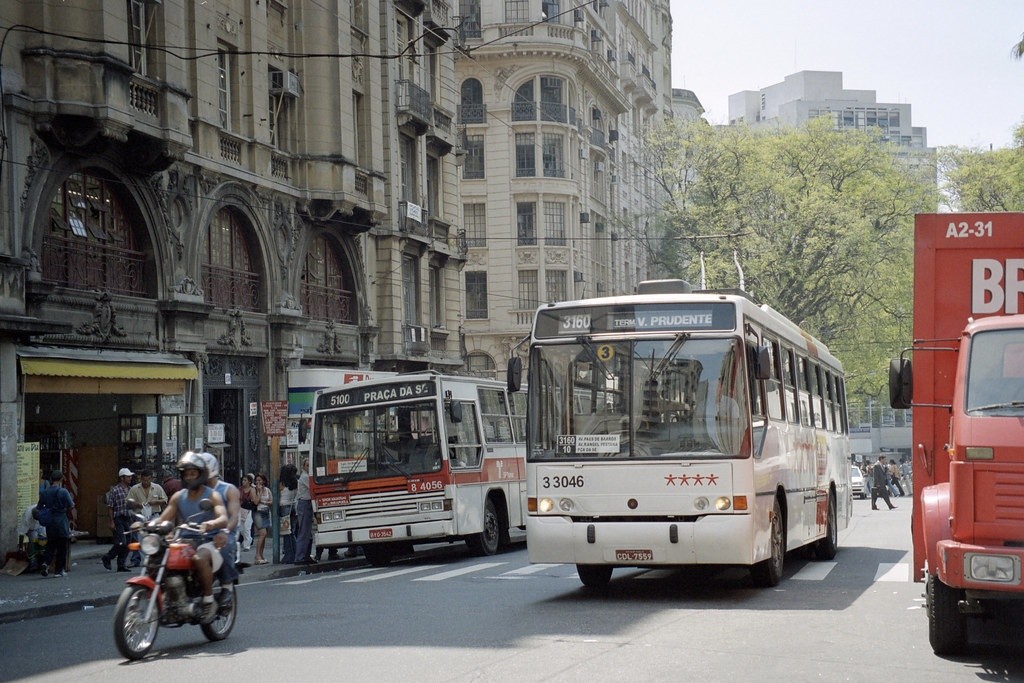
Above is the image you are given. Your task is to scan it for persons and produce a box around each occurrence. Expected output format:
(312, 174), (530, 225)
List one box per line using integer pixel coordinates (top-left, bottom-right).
(239, 458), (364, 565)
(162, 470), (183, 500)
(166, 451), (245, 608)
(102, 468), (135, 572)
(871, 454), (898, 510)
(855, 458), (913, 496)
(18, 466), (75, 578)
(125, 470), (168, 568)
(692, 372), (739, 449)
(130, 451), (229, 624)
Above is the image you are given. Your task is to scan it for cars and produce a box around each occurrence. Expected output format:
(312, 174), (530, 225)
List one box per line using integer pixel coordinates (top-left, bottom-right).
(892, 474), (913, 496)
(851, 465), (867, 499)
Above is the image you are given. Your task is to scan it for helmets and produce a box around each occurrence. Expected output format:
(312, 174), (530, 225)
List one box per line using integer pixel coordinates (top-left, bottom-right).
(174, 451), (209, 490)
(199, 453), (220, 479)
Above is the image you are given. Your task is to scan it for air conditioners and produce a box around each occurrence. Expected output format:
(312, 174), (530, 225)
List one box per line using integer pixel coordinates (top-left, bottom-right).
(597, 282), (607, 292)
(574, 271), (584, 282)
(595, 161), (605, 171)
(611, 175), (619, 183)
(580, 148), (589, 158)
(575, 9), (585, 21)
(270, 70), (300, 97)
(595, 222), (604, 233)
(612, 233), (618, 241)
(607, 49), (618, 62)
(593, 109), (602, 119)
(581, 213), (590, 223)
(610, 130), (618, 142)
(590, 29), (602, 39)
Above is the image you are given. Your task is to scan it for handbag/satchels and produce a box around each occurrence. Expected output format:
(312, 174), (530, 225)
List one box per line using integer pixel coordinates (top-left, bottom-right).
(279, 514), (292, 536)
(141, 503), (152, 521)
(241, 487), (256, 510)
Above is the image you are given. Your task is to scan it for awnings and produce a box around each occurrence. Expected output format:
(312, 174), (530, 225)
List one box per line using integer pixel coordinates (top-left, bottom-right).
(16, 345), (199, 380)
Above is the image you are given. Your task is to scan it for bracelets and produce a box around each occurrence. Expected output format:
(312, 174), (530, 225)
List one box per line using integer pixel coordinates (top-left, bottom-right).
(20, 548), (23, 551)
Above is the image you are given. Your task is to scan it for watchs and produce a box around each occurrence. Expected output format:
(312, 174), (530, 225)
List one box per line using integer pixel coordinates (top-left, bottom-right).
(221, 527), (230, 534)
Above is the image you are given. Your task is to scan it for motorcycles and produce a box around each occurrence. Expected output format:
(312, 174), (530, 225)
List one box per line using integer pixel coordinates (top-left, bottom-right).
(111, 498), (237, 660)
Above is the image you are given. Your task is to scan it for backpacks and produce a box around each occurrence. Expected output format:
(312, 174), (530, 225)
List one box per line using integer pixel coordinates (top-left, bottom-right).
(37, 487), (61, 527)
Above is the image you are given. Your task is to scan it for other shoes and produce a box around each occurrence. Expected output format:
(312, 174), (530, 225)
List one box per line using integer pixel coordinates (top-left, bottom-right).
(128, 563), (141, 568)
(328, 554), (345, 560)
(255, 558), (270, 564)
(53, 569), (68, 577)
(294, 557), (318, 564)
(313, 556), (321, 561)
(117, 566), (132, 572)
(102, 555), (112, 570)
(40, 563), (49, 577)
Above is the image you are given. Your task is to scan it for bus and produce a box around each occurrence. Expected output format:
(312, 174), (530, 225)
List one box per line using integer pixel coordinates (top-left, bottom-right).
(309, 370), (613, 555)
(506, 278), (853, 587)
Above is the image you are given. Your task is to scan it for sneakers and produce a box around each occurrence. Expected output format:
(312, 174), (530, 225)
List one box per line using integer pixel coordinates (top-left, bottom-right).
(216, 587), (232, 607)
(199, 600), (218, 625)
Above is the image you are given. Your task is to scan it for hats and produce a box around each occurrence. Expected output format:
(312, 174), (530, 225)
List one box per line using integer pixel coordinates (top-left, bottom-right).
(118, 468), (135, 477)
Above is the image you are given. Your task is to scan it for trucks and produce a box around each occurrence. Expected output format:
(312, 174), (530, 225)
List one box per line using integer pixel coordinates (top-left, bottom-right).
(889, 212), (1024, 653)
